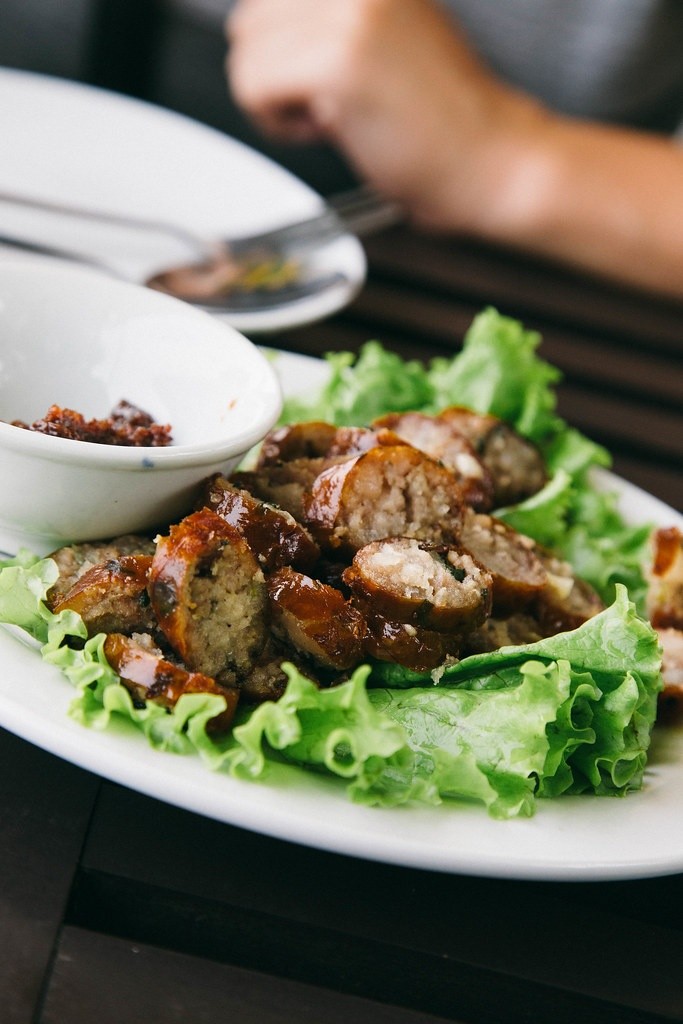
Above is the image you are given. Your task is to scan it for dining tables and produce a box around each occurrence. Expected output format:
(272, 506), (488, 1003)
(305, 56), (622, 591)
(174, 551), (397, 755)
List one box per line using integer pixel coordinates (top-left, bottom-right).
(0, 216), (683, 1024)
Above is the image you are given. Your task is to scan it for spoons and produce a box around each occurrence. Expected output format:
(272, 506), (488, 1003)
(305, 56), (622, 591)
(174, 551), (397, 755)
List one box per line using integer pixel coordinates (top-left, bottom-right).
(0, 235), (348, 309)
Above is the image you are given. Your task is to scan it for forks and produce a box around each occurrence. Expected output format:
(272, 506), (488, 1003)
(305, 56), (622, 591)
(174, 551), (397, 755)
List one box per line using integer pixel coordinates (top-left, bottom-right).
(6, 179), (405, 263)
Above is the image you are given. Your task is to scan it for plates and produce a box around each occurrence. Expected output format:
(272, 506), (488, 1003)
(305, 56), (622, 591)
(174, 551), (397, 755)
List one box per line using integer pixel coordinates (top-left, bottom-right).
(2, 68), (367, 335)
(2, 347), (682, 887)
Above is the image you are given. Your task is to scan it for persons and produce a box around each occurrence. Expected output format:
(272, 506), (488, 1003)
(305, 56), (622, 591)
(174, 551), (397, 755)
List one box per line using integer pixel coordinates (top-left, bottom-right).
(0, 0), (683, 296)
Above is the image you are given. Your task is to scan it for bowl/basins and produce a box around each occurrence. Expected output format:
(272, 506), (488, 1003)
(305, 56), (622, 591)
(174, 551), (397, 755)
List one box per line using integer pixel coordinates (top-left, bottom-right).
(1, 264), (287, 556)
